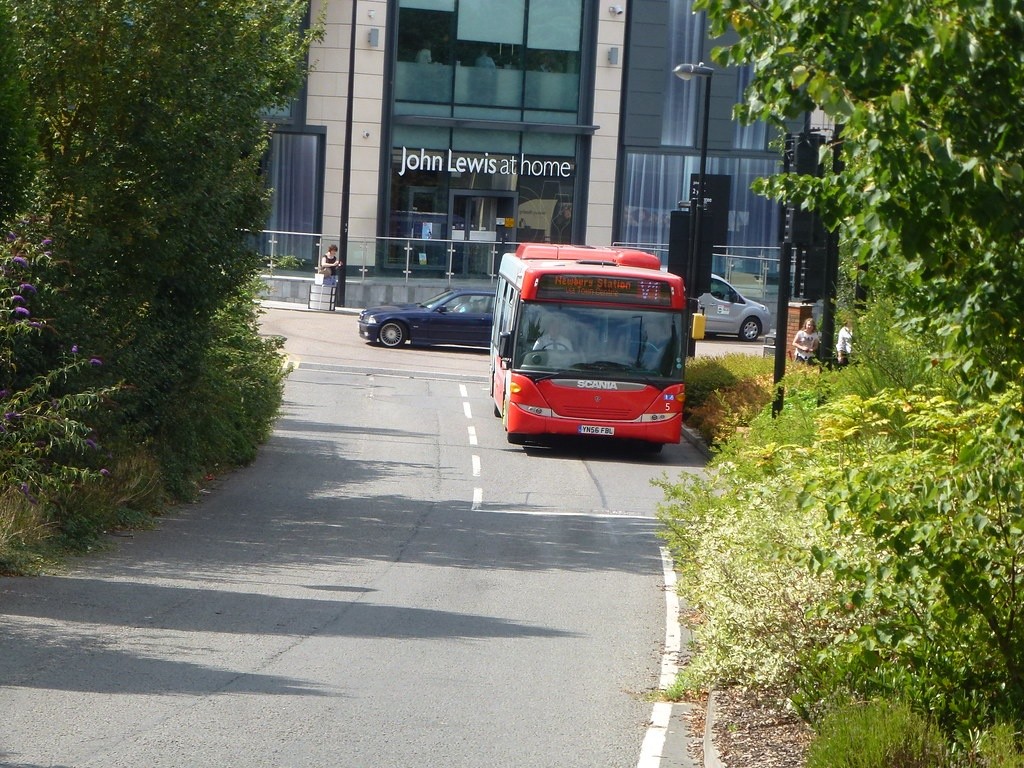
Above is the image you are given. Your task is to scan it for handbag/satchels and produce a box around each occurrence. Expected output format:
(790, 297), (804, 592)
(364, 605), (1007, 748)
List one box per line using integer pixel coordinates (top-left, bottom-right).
(318, 255), (332, 278)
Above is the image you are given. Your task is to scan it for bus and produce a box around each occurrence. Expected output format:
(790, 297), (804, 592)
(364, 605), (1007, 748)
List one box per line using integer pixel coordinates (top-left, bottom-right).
(489, 243), (706, 444)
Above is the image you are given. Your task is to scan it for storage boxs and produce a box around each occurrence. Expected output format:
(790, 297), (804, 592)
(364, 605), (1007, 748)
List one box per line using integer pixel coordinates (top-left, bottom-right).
(308, 301), (336, 311)
(309, 293), (336, 302)
(310, 284), (336, 295)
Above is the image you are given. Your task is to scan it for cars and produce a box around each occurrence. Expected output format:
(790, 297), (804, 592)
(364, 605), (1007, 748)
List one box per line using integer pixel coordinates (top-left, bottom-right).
(358, 288), (496, 350)
(660, 267), (771, 341)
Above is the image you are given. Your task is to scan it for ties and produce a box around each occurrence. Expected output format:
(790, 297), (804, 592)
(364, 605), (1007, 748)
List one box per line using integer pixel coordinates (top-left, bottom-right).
(552, 338), (558, 351)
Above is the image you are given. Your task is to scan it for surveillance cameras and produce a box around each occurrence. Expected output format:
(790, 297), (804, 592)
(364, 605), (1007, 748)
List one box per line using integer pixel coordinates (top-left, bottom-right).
(608, 3), (623, 14)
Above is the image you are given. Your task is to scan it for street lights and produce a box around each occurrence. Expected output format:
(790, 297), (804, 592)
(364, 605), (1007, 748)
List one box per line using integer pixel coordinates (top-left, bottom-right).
(671, 62), (715, 358)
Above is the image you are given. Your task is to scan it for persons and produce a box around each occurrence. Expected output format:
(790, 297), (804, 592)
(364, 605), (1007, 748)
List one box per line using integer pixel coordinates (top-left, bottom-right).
(791, 318), (818, 359)
(533, 320), (574, 352)
(443, 295), (472, 312)
(836, 322), (853, 368)
(322, 244), (344, 307)
